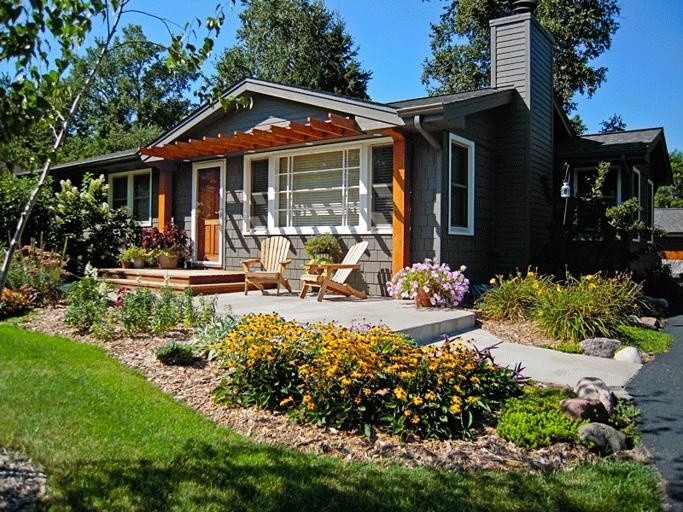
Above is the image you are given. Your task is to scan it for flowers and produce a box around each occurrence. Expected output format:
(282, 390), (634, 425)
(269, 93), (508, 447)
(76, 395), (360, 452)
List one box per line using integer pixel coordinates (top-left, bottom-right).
(385, 257), (471, 307)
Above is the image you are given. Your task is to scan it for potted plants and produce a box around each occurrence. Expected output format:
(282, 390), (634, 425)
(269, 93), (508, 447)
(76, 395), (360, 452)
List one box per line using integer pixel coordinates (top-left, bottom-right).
(305, 235), (342, 260)
(121, 239), (181, 268)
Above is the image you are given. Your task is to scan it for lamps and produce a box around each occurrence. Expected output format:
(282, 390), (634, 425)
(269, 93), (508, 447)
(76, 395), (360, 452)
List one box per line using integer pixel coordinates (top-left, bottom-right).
(561, 161), (572, 199)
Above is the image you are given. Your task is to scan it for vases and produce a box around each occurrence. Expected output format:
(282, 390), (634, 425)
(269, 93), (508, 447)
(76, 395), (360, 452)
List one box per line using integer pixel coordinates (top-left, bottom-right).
(414, 287), (438, 308)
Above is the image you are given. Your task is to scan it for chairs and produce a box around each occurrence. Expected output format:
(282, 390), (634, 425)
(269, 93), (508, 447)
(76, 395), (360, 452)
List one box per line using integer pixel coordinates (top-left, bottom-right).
(300, 240), (369, 302)
(240, 235), (292, 297)
